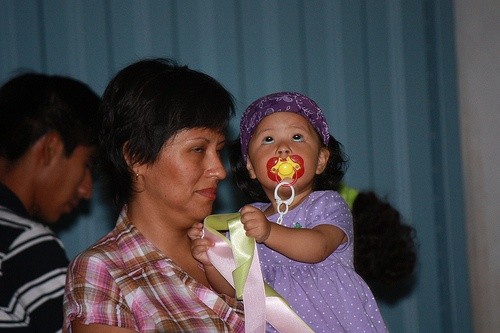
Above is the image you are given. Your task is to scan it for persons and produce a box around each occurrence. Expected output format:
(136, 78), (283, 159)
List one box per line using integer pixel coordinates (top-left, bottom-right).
(0, 73), (101, 333)
(187, 91), (387, 333)
(63, 58), (280, 333)
